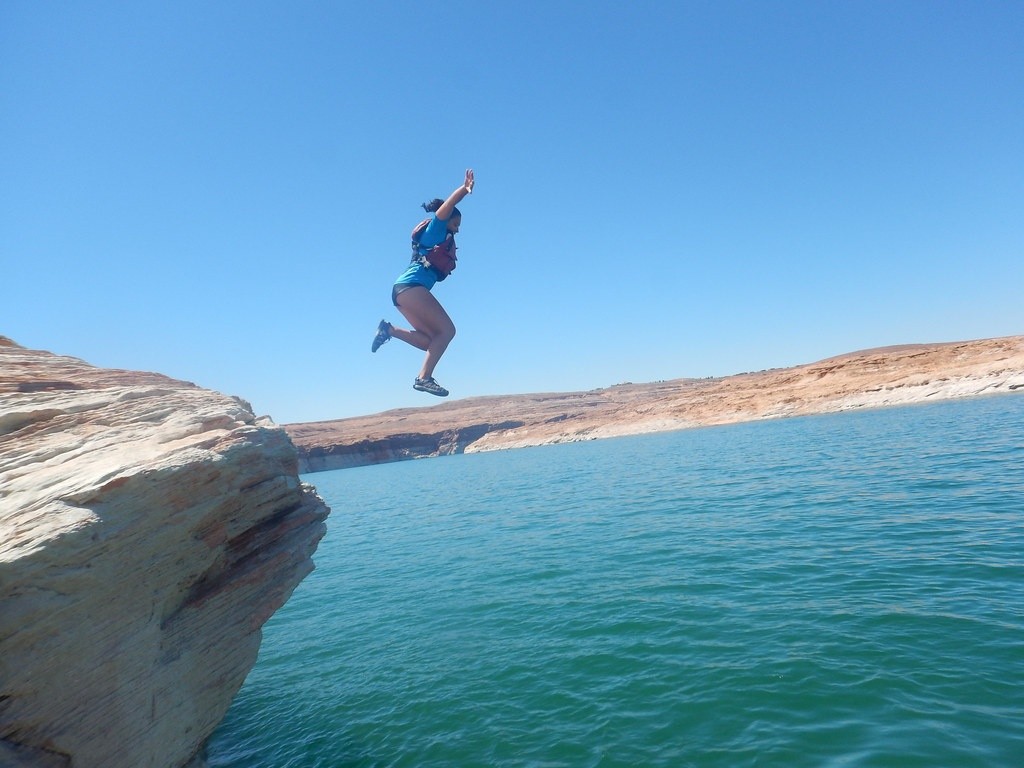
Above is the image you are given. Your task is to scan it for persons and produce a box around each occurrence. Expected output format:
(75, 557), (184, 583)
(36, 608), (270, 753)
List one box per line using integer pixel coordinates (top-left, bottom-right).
(372, 169), (475, 397)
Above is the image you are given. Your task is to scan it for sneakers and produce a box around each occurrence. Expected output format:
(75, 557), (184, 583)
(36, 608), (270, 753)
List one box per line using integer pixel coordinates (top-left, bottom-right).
(371, 320), (392, 352)
(413, 377), (449, 396)
